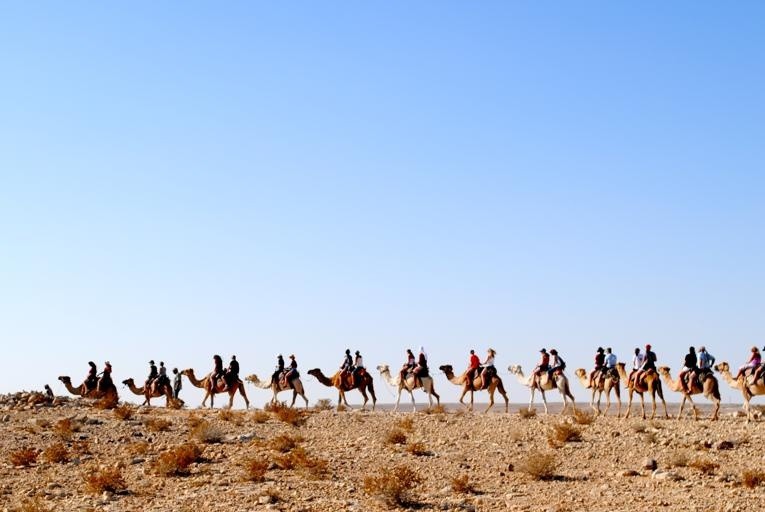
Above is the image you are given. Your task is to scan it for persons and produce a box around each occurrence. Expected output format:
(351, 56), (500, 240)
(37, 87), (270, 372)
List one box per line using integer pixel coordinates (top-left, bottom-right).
(44, 384), (54, 399)
